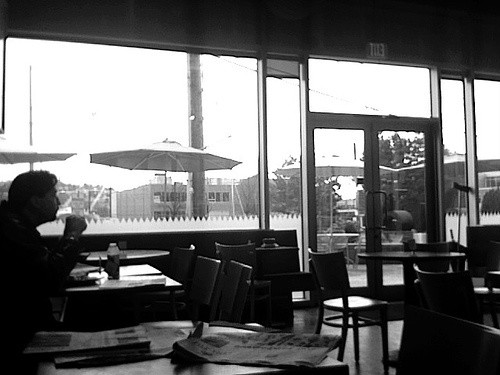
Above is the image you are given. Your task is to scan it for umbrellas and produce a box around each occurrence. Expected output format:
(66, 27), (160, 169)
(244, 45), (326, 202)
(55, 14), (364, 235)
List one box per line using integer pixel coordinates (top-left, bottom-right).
(90, 137), (243, 220)
(397, 150), (500, 243)
(272, 150), (397, 235)
(0, 128), (79, 167)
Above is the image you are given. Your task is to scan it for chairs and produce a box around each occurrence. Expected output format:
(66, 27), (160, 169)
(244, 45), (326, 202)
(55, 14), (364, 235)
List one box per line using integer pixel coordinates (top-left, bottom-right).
(308, 248), (390, 371)
(218, 260), (252, 324)
(397, 303), (500, 375)
(171, 248), (195, 278)
(193, 255), (222, 320)
(412, 263), (500, 328)
(218, 242), (256, 276)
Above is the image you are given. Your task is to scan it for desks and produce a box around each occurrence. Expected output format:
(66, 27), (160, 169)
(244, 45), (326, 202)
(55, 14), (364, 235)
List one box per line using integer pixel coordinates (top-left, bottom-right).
(86, 251), (170, 263)
(39, 323), (349, 375)
(65, 264), (183, 320)
(356, 251), (467, 308)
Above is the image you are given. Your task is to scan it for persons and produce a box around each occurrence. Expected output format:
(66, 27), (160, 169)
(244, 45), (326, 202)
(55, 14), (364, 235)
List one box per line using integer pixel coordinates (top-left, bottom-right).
(0, 170), (87, 375)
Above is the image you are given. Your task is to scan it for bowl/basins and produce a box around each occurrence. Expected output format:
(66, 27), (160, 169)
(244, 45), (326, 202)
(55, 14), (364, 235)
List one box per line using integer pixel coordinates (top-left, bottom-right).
(262, 238), (275, 245)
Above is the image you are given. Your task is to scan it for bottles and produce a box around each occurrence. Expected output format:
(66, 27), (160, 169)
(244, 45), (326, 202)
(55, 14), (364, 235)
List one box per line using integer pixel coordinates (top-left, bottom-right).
(106, 243), (120, 280)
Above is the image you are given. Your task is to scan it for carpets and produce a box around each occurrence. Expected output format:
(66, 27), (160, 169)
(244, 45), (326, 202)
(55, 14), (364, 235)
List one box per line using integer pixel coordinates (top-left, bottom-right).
(359, 301), (405, 321)
